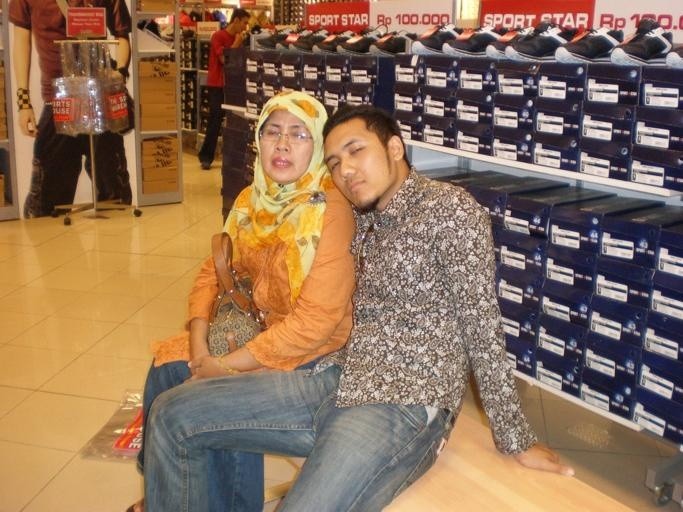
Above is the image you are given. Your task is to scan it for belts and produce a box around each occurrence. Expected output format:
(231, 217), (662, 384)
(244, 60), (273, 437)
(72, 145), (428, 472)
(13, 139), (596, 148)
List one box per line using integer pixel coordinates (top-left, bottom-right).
(445, 409), (457, 425)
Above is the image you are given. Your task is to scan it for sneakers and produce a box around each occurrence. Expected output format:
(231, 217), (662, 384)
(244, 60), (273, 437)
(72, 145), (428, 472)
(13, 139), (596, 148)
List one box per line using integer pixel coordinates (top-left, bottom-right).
(369, 31), (418, 57)
(487, 27), (535, 57)
(555, 26), (624, 63)
(290, 28), (329, 51)
(167, 29), (195, 39)
(336, 26), (383, 54)
(443, 26), (510, 57)
(505, 23), (577, 63)
(312, 30), (358, 53)
(611, 19), (672, 66)
(256, 28), (294, 49)
(665, 47), (683, 68)
(412, 23), (466, 54)
(202, 161), (210, 169)
(276, 30), (313, 50)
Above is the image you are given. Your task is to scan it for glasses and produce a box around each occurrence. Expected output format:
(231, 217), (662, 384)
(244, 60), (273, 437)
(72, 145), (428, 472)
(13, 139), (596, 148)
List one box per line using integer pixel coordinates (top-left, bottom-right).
(260, 128), (313, 143)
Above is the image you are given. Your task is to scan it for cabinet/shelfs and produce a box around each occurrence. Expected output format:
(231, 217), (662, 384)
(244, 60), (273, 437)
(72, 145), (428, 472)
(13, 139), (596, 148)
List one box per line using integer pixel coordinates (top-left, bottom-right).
(180, 1), (224, 160)
(130, 1), (182, 208)
(221, 45), (683, 451)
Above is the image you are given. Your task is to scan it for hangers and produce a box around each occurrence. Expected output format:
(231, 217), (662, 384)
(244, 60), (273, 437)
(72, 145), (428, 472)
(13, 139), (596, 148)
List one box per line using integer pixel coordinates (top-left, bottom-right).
(52, 38), (144, 226)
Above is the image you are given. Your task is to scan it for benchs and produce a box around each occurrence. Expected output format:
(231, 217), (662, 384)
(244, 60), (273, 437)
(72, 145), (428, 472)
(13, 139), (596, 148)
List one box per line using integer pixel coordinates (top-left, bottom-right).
(258, 410), (634, 512)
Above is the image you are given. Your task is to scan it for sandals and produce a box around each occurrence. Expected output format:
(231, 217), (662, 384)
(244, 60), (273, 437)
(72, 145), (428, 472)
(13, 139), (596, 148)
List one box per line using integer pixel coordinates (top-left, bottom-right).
(126, 499), (146, 512)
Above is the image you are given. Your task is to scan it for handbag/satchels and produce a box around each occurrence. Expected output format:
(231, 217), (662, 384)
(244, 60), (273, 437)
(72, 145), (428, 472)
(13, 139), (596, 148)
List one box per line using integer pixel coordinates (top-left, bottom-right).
(51, 43), (128, 136)
(207, 232), (265, 356)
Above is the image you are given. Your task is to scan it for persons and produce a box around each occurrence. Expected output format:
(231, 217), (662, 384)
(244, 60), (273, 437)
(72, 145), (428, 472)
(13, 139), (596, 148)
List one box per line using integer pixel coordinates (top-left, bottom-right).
(198, 8), (251, 170)
(8, 0), (132, 218)
(143, 104), (574, 512)
(128, 90), (357, 511)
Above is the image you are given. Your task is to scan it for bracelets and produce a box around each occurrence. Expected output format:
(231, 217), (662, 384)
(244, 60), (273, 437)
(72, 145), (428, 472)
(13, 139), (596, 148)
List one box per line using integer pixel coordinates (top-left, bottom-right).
(216, 354), (242, 375)
